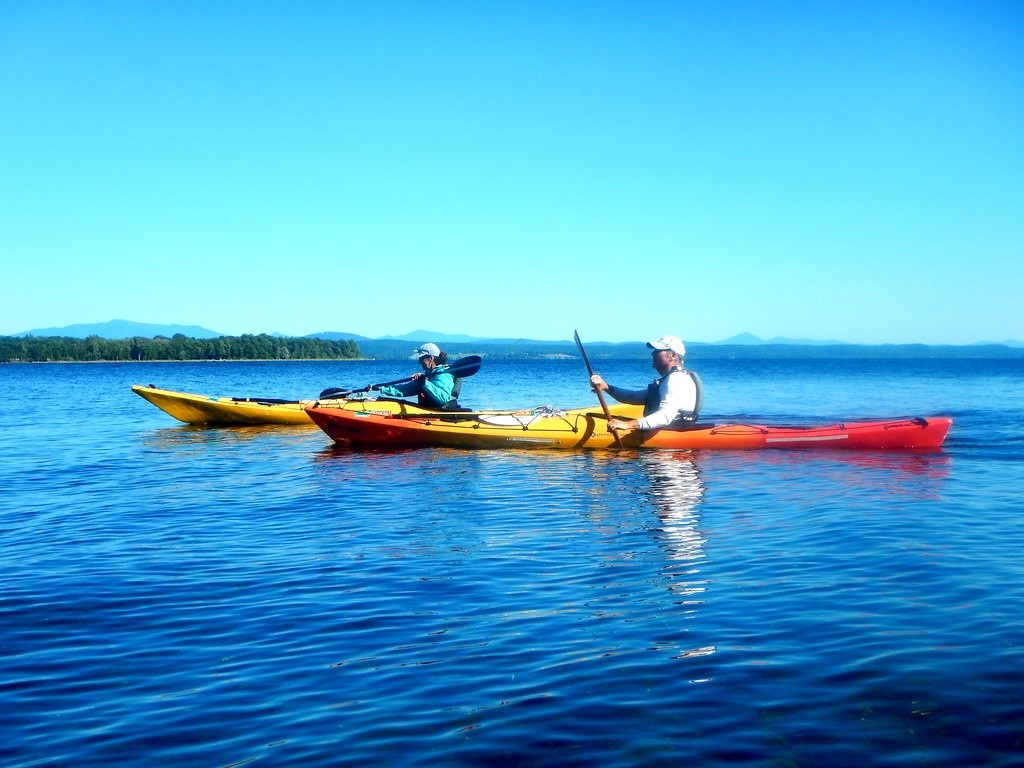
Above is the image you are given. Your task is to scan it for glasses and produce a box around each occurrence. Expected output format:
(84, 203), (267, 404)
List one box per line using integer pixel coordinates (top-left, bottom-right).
(417, 349), (430, 354)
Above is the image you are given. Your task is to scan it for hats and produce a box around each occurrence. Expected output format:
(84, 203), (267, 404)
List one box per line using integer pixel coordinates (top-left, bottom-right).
(410, 343), (441, 359)
(646, 336), (686, 357)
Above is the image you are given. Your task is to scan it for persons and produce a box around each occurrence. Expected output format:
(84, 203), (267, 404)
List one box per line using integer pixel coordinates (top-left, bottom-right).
(590, 335), (696, 433)
(369, 343), (462, 409)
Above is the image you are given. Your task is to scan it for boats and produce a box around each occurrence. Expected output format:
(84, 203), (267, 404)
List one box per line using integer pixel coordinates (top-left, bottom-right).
(302, 400), (953, 452)
(132, 385), (645, 426)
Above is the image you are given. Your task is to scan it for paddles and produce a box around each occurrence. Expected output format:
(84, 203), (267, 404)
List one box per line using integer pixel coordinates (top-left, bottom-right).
(319, 355), (481, 401)
(573, 329), (624, 450)
